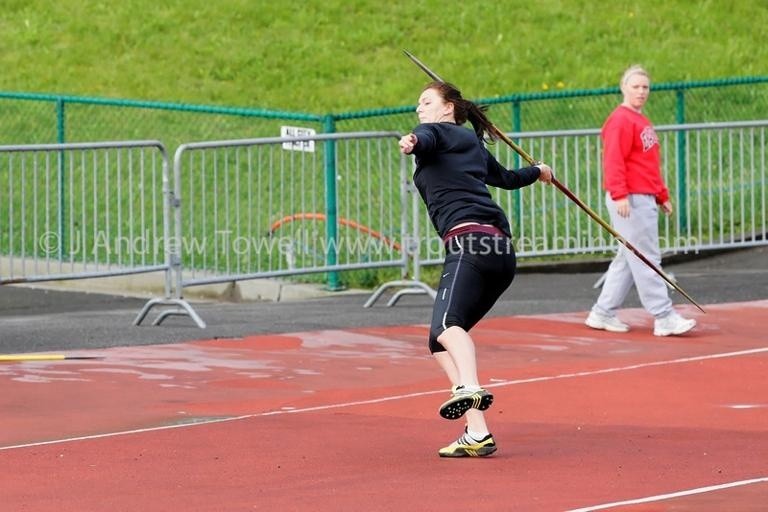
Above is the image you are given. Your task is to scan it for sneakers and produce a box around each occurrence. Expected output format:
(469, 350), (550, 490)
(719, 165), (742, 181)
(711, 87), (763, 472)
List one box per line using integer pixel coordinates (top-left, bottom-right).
(438, 423), (497, 456)
(585, 311), (630, 334)
(437, 384), (494, 419)
(652, 310), (697, 337)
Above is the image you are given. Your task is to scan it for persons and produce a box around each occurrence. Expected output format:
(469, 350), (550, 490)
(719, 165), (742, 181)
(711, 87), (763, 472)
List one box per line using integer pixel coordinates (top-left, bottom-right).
(583, 62), (698, 337)
(396, 79), (556, 460)
